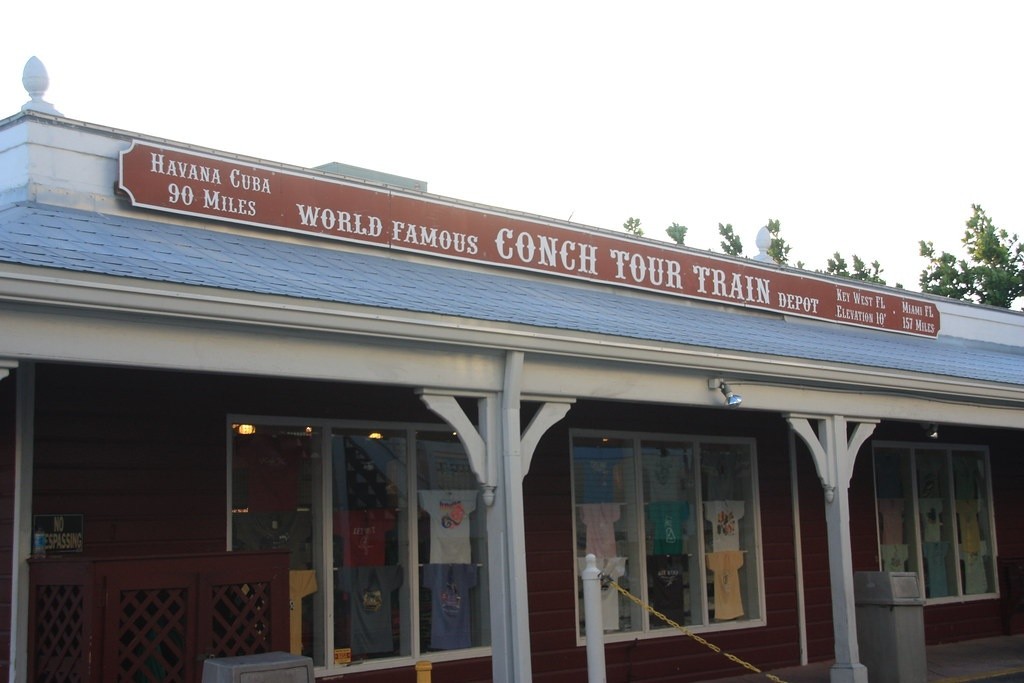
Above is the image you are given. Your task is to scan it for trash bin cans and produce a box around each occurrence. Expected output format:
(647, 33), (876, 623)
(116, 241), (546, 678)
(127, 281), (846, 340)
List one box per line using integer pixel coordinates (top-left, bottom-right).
(200, 650), (316, 683)
(854, 570), (929, 683)
